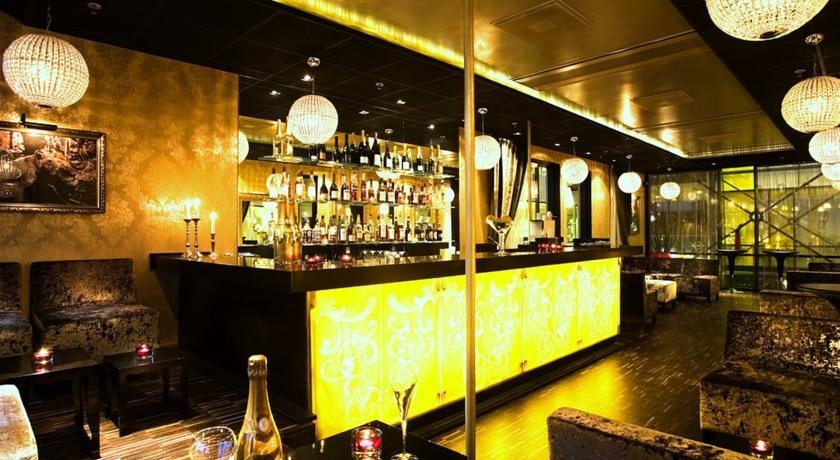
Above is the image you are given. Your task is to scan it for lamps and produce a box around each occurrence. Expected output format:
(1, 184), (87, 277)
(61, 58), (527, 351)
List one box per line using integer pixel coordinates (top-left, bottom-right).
(560, 138), (589, 185)
(618, 154), (642, 194)
(781, 33), (840, 190)
(705, 0), (829, 41)
(474, 107), (501, 171)
(288, 56), (338, 147)
(658, 167), (681, 200)
(2, 7), (90, 109)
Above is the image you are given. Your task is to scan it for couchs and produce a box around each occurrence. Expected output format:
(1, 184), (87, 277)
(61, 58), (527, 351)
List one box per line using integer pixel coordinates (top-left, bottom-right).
(0, 258), (162, 372)
(547, 256), (840, 460)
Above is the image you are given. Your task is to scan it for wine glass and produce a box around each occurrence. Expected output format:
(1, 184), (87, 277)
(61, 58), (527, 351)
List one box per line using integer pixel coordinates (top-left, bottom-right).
(389, 361), (419, 460)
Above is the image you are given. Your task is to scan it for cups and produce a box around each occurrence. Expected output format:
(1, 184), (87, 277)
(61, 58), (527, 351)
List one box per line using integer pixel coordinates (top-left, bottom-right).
(749, 436), (774, 460)
(189, 426), (238, 460)
(31, 342), (53, 372)
(350, 426), (383, 460)
(538, 238), (562, 254)
(135, 334), (154, 360)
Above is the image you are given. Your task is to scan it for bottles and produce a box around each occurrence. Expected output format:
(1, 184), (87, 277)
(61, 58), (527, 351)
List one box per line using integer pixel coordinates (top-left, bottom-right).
(273, 193), (302, 266)
(233, 354), (284, 460)
(255, 116), (446, 244)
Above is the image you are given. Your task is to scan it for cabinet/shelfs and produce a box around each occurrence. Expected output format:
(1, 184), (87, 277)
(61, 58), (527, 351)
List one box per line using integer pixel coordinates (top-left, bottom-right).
(238, 155), (455, 259)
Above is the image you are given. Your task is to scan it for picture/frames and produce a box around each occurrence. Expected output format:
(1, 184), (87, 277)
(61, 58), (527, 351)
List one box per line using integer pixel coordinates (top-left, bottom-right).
(0, 121), (106, 215)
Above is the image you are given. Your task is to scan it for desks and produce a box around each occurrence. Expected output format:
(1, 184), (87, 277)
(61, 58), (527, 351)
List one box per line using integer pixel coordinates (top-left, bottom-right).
(0, 344), (193, 460)
(282, 420), (469, 460)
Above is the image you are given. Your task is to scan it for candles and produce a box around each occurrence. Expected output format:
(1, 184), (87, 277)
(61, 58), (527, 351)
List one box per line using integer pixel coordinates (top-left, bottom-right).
(211, 214), (216, 233)
(354, 429), (382, 452)
(136, 345), (153, 357)
(33, 347), (54, 363)
(185, 200), (199, 218)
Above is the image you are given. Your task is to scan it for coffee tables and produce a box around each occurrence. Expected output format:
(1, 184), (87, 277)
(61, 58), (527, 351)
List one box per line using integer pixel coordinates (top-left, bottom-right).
(796, 282), (840, 297)
(715, 248), (748, 294)
(645, 279), (677, 312)
(761, 249), (800, 291)
